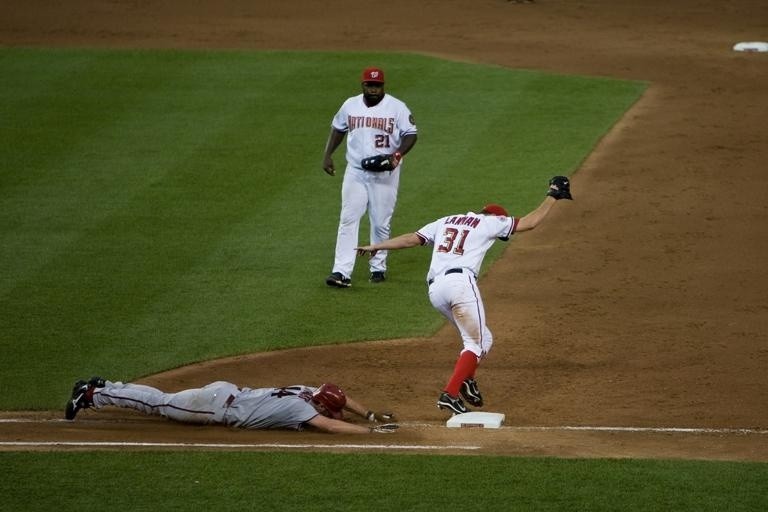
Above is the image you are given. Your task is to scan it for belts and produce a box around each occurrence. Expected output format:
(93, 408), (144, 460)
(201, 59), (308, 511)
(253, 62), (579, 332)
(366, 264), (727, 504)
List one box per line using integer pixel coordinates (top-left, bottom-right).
(429, 268), (462, 285)
(226, 388), (241, 406)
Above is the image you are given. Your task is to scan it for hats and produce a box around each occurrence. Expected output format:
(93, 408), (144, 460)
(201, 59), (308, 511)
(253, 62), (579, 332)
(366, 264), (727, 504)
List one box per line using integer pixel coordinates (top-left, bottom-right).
(361, 68), (384, 83)
(482, 205), (509, 241)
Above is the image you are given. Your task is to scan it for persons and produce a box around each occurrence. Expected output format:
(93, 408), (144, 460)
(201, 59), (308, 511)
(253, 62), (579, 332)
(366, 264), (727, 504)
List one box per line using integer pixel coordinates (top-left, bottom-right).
(64, 373), (402, 436)
(353, 173), (574, 414)
(321, 67), (420, 289)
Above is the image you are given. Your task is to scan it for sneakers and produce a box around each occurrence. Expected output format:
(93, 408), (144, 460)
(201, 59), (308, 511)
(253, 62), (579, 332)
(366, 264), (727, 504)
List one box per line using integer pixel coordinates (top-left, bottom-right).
(369, 271), (386, 282)
(327, 272), (352, 287)
(459, 377), (483, 407)
(437, 393), (470, 414)
(65, 381), (93, 421)
(89, 377), (105, 388)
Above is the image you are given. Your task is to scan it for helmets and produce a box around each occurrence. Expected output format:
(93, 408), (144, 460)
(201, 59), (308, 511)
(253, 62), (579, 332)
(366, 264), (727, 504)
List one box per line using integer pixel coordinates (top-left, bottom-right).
(312, 384), (346, 419)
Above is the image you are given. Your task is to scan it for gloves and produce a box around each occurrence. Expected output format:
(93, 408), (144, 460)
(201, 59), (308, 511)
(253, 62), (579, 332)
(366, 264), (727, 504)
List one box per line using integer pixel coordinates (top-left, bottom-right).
(368, 424), (399, 434)
(365, 410), (395, 423)
